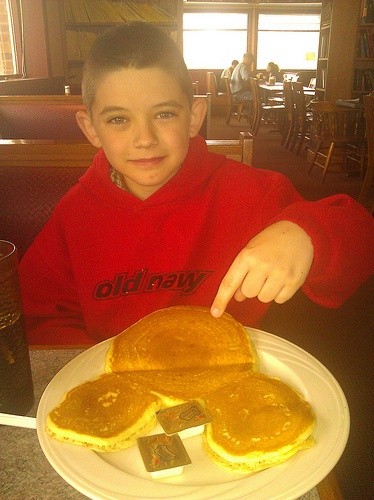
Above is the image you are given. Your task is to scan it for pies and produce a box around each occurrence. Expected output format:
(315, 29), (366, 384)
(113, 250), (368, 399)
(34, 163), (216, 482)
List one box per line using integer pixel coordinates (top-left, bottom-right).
(104, 306), (256, 403)
(203, 373), (317, 466)
(46, 374), (163, 452)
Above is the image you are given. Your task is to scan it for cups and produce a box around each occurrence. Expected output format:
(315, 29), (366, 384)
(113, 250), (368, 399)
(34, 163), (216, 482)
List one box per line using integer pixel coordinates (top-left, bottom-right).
(0, 239), (35, 426)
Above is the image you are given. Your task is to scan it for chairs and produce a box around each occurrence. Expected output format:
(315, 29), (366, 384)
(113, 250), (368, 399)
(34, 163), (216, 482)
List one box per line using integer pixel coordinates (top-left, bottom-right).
(194, 66), (374, 199)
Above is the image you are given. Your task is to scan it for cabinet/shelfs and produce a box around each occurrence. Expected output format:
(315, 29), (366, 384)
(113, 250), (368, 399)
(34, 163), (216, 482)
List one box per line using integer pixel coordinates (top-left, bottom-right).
(316, 0), (360, 100)
(353, 0), (374, 100)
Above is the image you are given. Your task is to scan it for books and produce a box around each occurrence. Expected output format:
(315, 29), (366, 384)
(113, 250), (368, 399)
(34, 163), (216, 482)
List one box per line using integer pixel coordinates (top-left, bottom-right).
(354, 0), (374, 91)
(318, 0), (333, 88)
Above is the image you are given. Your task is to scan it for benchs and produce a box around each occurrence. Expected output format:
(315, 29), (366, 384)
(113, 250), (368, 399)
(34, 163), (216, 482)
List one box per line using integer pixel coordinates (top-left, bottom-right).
(0, 132), (254, 261)
(0, 94), (212, 140)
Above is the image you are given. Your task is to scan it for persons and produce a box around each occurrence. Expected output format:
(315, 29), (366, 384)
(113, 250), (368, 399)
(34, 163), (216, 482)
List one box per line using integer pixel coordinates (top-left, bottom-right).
(220, 52), (283, 120)
(19, 20), (374, 348)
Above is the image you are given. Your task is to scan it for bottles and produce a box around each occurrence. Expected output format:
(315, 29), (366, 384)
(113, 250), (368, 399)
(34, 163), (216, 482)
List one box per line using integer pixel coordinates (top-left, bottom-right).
(64, 85), (71, 96)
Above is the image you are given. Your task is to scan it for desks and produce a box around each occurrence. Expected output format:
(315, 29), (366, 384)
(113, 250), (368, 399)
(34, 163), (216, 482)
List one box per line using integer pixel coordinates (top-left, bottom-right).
(310, 99), (365, 170)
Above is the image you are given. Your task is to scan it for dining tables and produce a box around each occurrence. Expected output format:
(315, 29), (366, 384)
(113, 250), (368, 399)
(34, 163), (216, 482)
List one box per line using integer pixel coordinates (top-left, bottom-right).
(0, 349), (344, 500)
(258, 81), (315, 120)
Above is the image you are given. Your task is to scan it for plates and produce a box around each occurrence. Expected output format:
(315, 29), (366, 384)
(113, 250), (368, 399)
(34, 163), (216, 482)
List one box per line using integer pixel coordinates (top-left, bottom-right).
(35, 327), (350, 500)
